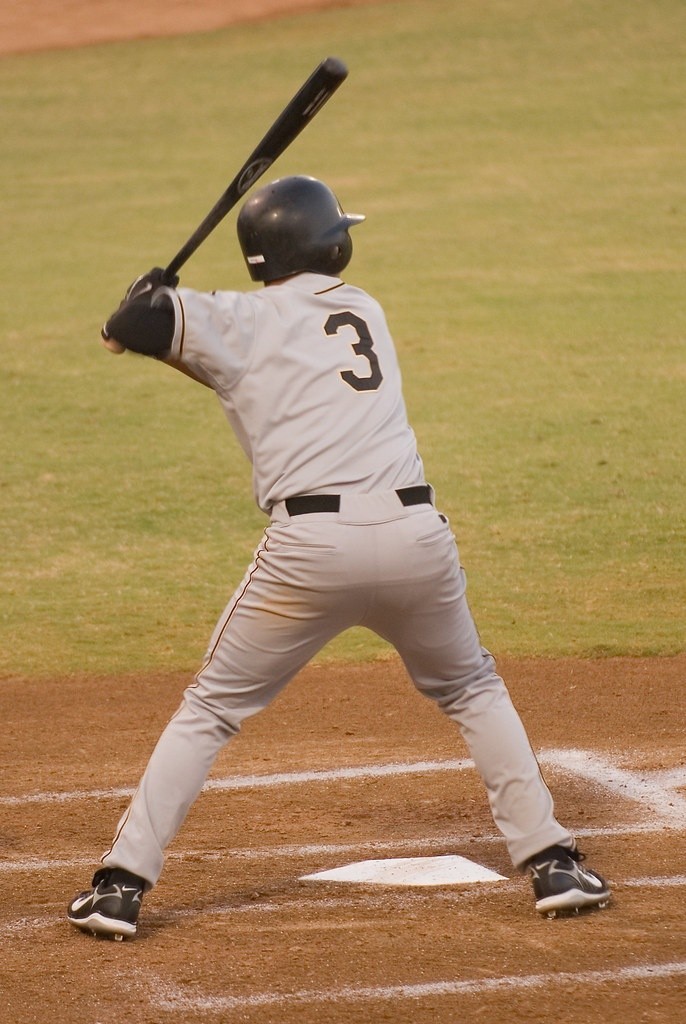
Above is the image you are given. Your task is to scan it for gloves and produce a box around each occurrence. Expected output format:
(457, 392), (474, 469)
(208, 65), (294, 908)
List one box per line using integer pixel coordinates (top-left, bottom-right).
(101, 267), (180, 341)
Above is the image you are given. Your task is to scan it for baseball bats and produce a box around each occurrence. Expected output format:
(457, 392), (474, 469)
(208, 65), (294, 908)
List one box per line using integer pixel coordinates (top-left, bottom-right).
(101, 57), (351, 355)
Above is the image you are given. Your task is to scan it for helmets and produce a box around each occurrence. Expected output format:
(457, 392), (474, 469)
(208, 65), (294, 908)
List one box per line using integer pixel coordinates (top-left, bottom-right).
(237, 175), (366, 281)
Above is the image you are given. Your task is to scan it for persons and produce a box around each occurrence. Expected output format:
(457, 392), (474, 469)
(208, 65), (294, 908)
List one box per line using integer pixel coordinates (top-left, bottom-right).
(69, 175), (611, 943)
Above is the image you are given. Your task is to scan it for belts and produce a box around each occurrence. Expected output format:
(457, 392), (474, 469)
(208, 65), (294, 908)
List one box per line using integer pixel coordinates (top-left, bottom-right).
(268, 486), (433, 516)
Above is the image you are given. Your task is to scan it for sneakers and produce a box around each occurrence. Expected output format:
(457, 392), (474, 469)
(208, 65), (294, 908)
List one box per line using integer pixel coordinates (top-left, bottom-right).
(532, 845), (611, 918)
(67, 868), (143, 941)
